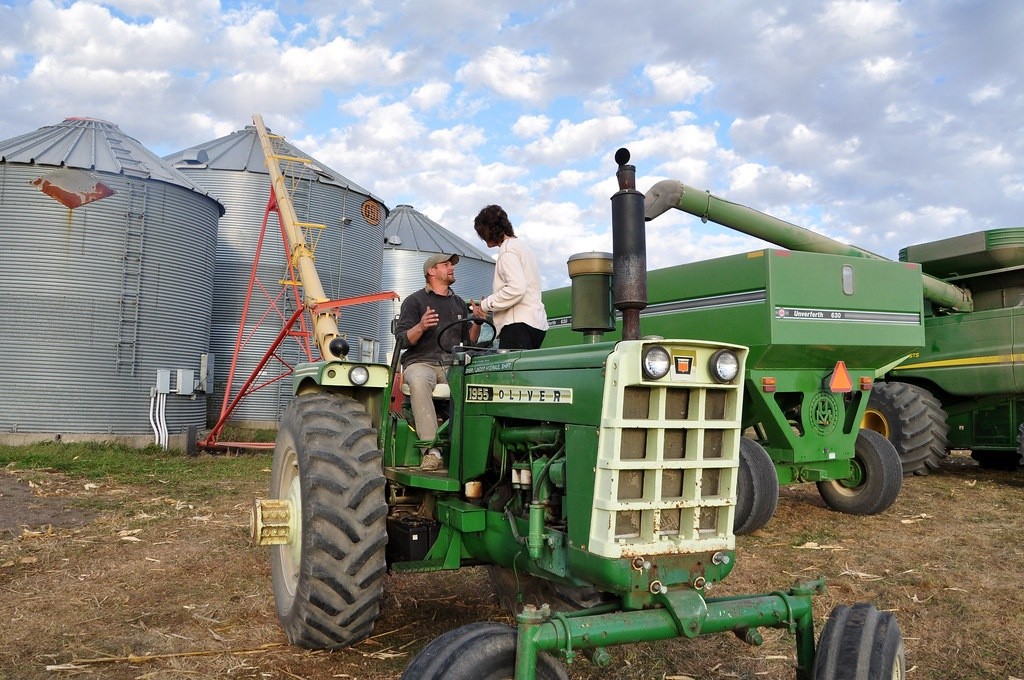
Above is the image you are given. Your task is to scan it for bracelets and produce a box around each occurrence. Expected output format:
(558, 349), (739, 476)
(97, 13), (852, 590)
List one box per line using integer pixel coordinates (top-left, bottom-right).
(473, 315), (483, 325)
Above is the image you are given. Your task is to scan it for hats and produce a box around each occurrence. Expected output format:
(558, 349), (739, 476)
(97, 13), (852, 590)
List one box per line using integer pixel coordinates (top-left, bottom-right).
(423, 253), (460, 277)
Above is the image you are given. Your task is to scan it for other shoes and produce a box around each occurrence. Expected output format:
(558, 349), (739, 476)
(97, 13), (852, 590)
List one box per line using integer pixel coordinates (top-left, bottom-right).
(420, 452), (444, 470)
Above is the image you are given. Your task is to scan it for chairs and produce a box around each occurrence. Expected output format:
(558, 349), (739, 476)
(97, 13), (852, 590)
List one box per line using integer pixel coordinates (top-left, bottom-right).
(391, 312), (453, 401)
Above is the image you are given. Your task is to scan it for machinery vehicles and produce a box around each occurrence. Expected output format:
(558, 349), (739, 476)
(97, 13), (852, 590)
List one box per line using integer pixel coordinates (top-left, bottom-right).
(250, 147), (908, 680)
(645, 179), (1024, 477)
(541, 250), (925, 536)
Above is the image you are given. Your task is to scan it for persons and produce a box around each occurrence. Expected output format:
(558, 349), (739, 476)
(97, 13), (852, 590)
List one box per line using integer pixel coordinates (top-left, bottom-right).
(395, 254), (487, 471)
(474, 205), (550, 352)
